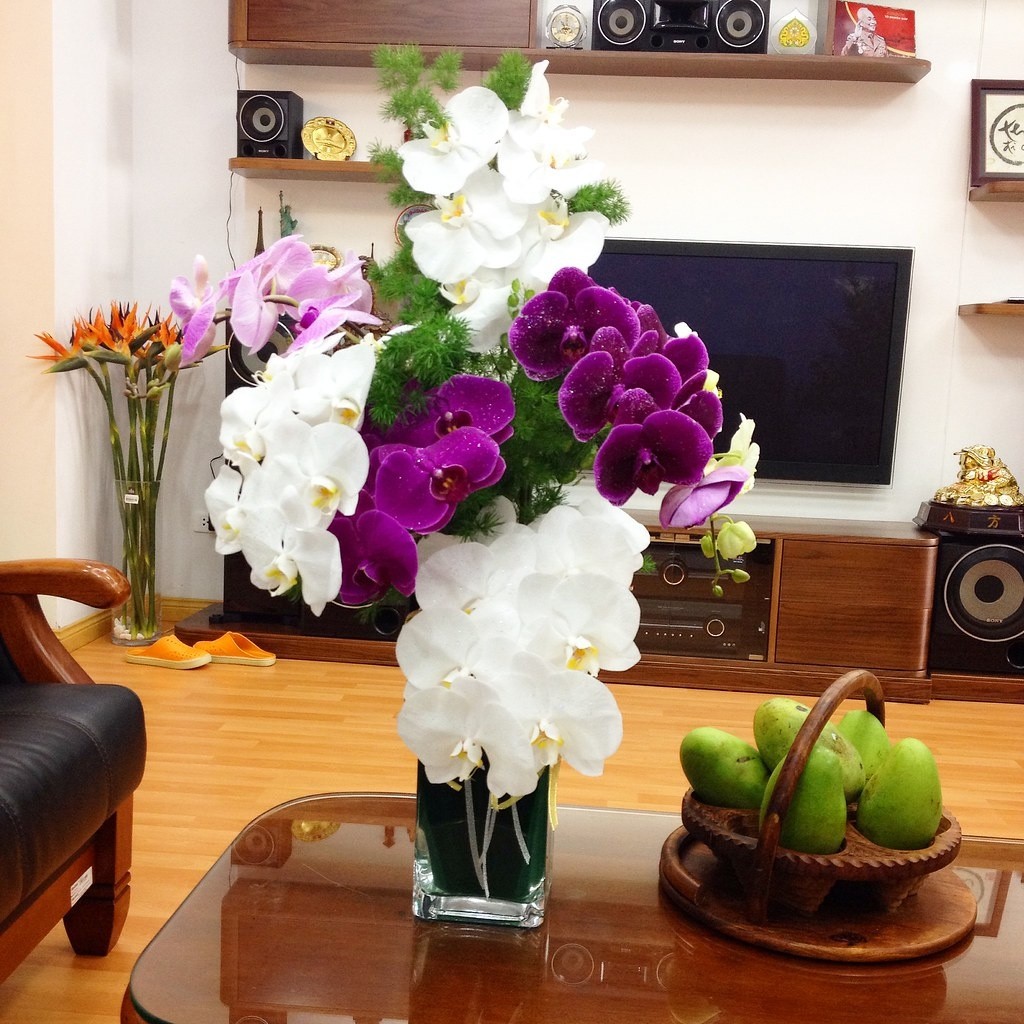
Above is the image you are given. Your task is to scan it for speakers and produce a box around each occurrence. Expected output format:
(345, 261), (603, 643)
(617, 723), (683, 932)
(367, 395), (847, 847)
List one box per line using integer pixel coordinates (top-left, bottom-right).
(298, 597), (415, 642)
(712, 0), (771, 55)
(926, 527), (1024, 677)
(236, 89), (306, 161)
(592, 0), (652, 51)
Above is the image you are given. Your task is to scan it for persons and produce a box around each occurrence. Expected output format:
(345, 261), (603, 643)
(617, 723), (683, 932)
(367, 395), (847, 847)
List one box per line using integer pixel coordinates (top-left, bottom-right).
(840, 8), (888, 58)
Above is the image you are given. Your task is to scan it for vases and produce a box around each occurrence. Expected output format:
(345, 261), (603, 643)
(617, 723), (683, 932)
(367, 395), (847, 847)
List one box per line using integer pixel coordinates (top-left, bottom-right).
(412, 753), (555, 933)
(111, 479), (161, 646)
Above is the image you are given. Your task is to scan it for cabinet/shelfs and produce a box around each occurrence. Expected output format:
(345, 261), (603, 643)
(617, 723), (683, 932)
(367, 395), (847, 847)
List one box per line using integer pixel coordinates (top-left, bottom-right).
(171, 508), (1024, 705)
(226, 1), (1024, 317)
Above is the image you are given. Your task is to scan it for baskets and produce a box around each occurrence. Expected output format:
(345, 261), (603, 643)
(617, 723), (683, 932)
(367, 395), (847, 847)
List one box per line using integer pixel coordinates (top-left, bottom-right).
(681, 668), (962, 919)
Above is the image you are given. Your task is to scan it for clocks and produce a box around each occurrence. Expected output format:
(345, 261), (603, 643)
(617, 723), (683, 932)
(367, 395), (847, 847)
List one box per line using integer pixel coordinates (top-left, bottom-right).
(544, 4), (587, 50)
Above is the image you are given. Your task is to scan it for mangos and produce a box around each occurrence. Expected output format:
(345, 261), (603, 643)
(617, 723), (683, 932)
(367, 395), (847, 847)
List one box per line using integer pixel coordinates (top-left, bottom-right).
(679, 697), (942, 855)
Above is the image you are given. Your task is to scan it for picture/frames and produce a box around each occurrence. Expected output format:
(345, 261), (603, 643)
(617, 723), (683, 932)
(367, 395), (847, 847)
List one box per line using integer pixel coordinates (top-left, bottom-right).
(971, 78), (1024, 187)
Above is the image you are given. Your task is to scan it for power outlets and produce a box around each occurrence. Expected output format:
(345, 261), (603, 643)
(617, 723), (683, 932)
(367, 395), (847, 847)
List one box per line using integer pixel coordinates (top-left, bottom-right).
(193, 512), (215, 533)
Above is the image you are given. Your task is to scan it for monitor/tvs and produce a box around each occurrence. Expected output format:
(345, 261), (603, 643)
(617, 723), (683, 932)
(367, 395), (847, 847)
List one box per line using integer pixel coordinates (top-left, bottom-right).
(589, 236), (916, 489)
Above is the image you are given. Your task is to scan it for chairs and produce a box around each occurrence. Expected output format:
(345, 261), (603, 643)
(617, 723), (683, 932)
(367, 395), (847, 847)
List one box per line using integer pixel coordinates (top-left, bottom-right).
(0, 559), (147, 985)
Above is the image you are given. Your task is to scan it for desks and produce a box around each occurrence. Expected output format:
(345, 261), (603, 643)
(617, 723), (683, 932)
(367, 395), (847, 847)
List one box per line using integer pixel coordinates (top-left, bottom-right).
(121, 792), (1024, 1024)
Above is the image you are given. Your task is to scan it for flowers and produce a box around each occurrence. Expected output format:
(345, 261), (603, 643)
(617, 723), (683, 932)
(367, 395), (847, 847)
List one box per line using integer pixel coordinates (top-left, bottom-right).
(26, 301), (228, 639)
